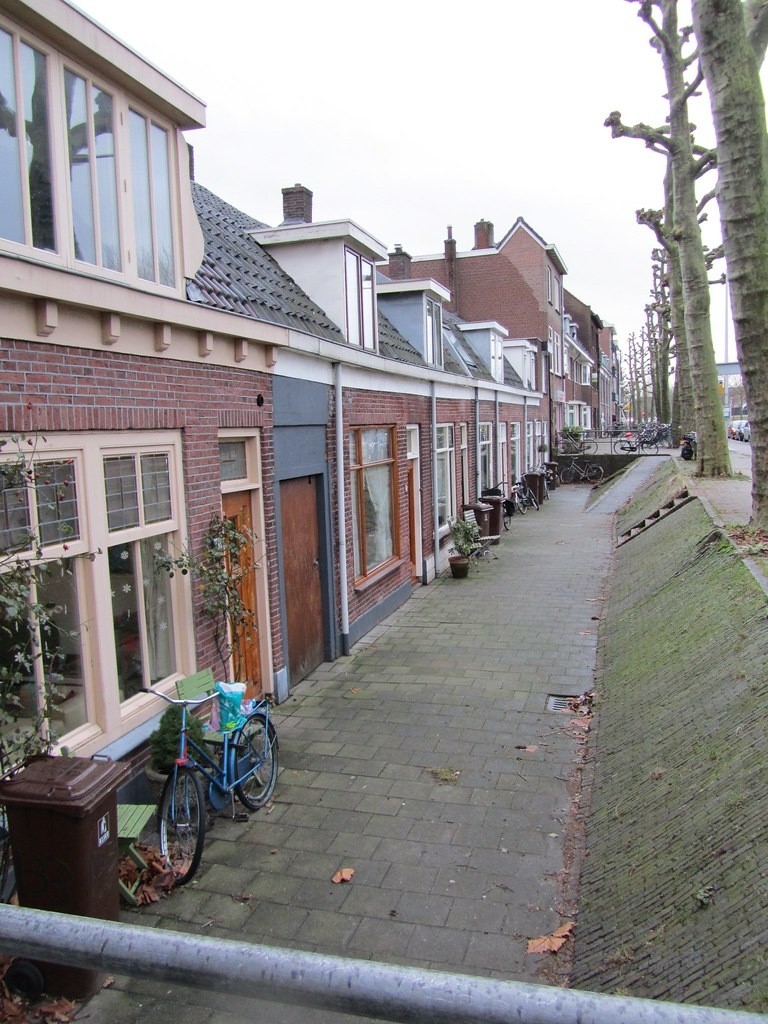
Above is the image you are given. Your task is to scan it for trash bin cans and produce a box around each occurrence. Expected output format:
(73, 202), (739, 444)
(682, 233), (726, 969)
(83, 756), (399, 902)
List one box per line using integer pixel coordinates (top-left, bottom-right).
(0, 751), (133, 1002)
(543, 462), (558, 490)
(522, 472), (545, 505)
(460, 503), (494, 550)
(478, 495), (506, 545)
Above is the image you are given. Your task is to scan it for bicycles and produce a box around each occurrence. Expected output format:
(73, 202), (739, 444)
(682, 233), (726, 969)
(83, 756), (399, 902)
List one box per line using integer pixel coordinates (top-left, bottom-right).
(560, 456), (604, 484)
(137, 681), (280, 887)
(482, 472), (539, 531)
(556, 419), (673, 454)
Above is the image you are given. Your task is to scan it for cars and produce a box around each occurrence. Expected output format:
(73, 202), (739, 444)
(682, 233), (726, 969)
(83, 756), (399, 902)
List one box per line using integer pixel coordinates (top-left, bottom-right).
(727, 420), (751, 442)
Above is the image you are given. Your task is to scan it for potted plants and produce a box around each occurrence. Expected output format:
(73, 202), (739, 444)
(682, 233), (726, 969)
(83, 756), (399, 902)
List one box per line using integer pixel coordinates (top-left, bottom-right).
(447, 515), (482, 579)
(144, 703), (221, 810)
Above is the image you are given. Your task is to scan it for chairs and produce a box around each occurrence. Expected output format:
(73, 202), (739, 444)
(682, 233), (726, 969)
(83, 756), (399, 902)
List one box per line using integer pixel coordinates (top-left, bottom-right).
(447, 510), (501, 573)
(176, 668), (265, 802)
(60, 746), (159, 906)
